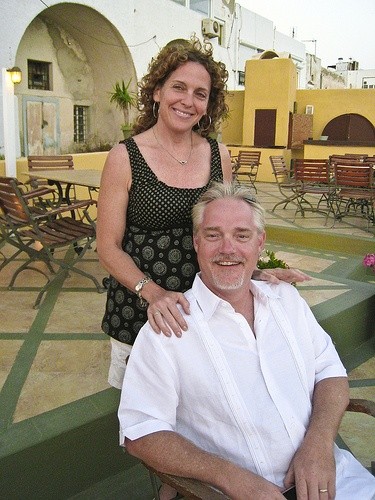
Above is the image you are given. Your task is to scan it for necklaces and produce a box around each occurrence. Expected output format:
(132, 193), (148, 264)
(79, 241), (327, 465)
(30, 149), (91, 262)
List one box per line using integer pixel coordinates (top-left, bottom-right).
(152, 126), (194, 166)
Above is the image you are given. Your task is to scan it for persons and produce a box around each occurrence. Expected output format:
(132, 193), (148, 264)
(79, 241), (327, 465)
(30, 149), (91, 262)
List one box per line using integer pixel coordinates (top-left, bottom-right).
(117, 179), (375, 500)
(96, 36), (312, 500)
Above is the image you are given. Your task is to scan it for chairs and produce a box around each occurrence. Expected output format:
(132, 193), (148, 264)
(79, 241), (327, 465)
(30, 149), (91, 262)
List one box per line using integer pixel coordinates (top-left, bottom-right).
(269, 152), (375, 233)
(228, 149), (261, 194)
(0, 155), (105, 310)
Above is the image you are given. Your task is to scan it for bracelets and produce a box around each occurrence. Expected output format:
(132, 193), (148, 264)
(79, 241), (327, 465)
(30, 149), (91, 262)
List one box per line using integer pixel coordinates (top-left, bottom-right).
(135, 276), (153, 308)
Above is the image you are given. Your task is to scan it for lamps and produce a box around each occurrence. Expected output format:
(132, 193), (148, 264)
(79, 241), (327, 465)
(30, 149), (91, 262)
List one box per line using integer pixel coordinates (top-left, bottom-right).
(6, 66), (23, 85)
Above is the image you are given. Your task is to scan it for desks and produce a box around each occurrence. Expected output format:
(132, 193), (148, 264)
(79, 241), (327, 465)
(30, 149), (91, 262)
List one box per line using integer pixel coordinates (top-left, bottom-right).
(21, 168), (103, 256)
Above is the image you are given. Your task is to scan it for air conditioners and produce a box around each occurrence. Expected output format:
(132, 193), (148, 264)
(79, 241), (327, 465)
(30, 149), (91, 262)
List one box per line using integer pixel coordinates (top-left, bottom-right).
(202, 18), (220, 39)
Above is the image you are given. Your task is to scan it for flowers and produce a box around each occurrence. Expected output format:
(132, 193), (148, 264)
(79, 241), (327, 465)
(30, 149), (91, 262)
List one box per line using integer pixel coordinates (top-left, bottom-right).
(363, 253), (375, 271)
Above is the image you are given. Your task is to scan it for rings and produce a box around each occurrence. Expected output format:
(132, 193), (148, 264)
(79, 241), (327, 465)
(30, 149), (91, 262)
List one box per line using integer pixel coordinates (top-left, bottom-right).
(319, 488), (328, 493)
